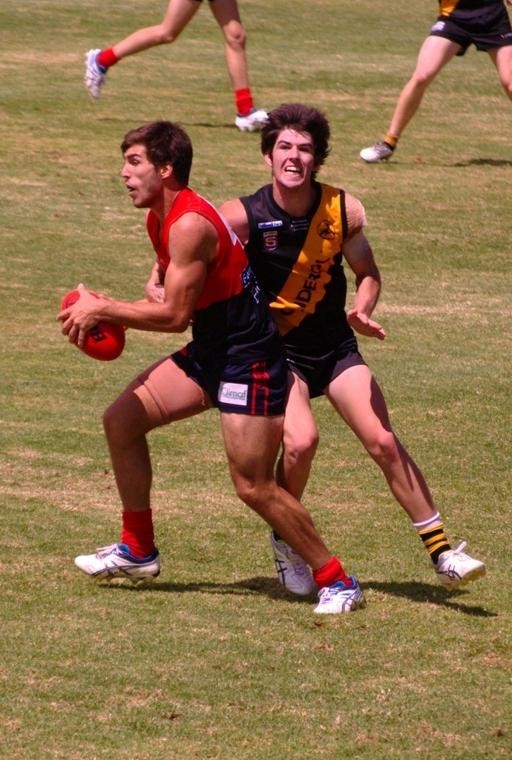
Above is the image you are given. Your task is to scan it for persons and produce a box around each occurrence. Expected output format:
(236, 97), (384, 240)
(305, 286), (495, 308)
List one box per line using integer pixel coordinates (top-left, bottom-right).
(142, 102), (490, 600)
(360, 0), (511, 162)
(85, 0), (273, 133)
(52, 119), (369, 620)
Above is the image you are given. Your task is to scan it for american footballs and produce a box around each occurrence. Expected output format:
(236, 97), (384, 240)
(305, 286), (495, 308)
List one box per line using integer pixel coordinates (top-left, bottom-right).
(61, 289), (125, 360)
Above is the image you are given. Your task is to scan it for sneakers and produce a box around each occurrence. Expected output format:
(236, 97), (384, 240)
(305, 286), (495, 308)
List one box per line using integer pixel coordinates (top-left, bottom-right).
(84, 48), (106, 99)
(269, 529), (315, 597)
(235, 109), (270, 132)
(75, 542), (161, 583)
(429, 540), (488, 592)
(312, 576), (367, 615)
(359, 141), (393, 163)
